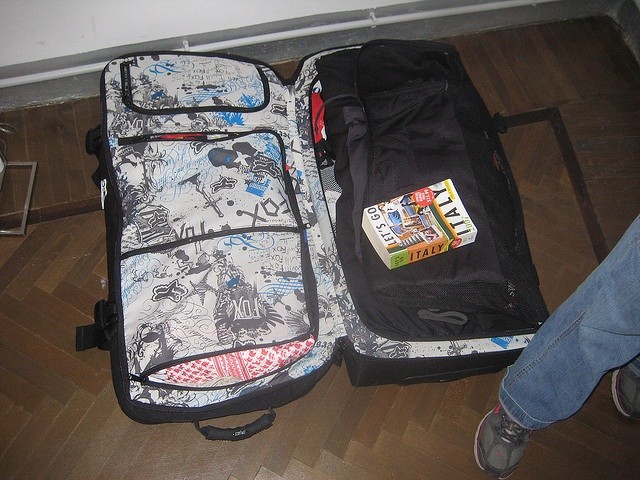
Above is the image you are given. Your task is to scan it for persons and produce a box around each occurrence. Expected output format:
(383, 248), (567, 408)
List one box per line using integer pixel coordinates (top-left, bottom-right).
(473, 214), (640, 480)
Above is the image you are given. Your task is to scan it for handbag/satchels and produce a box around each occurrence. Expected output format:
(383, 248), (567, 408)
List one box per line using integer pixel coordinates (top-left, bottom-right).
(75, 38), (551, 442)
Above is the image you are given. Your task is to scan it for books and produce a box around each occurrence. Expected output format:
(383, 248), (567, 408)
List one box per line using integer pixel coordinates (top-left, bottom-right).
(362, 178), (478, 270)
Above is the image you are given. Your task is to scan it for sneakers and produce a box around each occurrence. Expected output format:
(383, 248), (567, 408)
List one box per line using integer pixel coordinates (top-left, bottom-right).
(611, 358), (640, 420)
(473, 403), (532, 479)
